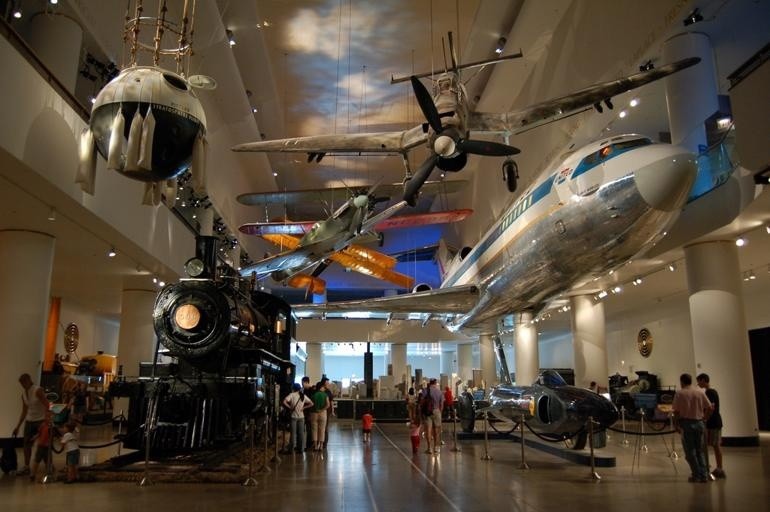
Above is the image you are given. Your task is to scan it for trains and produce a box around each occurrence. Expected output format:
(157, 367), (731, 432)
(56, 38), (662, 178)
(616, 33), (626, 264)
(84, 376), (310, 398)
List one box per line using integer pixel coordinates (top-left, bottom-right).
(45, 351), (118, 414)
(112, 233), (305, 460)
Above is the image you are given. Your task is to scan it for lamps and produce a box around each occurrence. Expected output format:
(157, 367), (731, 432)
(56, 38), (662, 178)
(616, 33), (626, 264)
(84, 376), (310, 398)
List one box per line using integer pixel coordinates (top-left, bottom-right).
(526, 299), (572, 330)
(740, 269), (758, 282)
(591, 261), (677, 308)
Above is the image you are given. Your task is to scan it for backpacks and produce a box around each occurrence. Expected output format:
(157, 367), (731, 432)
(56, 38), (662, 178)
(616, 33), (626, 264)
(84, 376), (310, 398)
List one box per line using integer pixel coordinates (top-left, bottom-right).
(420, 395), (435, 417)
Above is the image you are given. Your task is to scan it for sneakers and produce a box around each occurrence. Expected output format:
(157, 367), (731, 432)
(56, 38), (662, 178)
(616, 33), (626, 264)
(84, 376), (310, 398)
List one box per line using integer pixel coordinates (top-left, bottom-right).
(362, 438), (372, 442)
(290, 446), (324, 454)
(688, 469), (727, 483)
(411, 446), (441, 455)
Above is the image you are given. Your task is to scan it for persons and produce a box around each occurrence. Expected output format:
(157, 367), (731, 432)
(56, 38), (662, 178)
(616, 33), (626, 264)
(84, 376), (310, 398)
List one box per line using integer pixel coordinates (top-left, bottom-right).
(588, 381), (597, 390)
(31, 421), (50, 481)
(672, 373), (712, 483)
(11, 373), (55, 475)
(406, 379), (454, 454)
(283, 376), (334, 454)
(61, 423), (81, 484)
(696, 374), (726, 478)
(362, 408), (373, 442)
